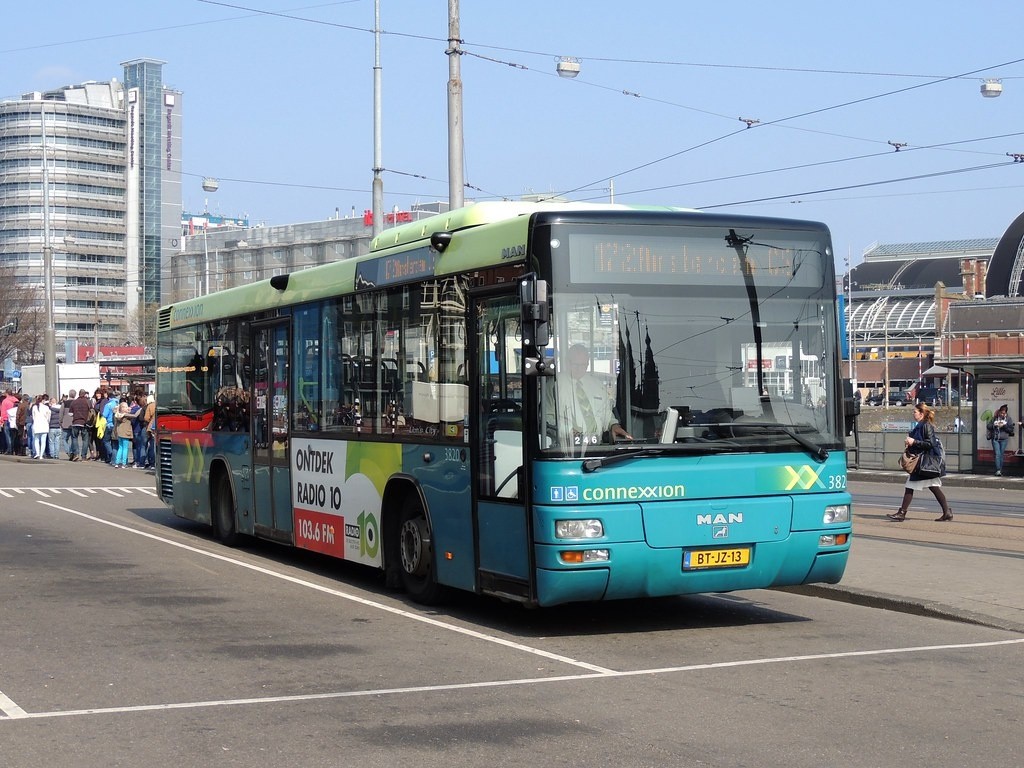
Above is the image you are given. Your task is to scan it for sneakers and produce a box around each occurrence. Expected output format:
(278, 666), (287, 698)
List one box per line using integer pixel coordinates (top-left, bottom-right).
(1, 446), (156, 471)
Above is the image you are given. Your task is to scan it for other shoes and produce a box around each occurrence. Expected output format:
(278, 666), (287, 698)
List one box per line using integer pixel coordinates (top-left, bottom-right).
(995, 469), (1001, 476)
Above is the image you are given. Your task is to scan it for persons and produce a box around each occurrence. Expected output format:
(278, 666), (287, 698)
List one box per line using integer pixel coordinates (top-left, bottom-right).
(674, 350), (729, 412)
(986, 404), (1014, 476)
(886, 401), (954, 522)
(0, 385), (156, 470)
(954, 415), (965, 434)
(853, 351), (923, 408)
(936, 396), (943, 406)
(539, 343), (634, 445)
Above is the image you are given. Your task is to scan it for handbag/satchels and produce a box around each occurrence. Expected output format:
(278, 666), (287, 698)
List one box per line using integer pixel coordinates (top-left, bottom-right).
(897, 447), (921, 474)
(919, 435), (945, 474)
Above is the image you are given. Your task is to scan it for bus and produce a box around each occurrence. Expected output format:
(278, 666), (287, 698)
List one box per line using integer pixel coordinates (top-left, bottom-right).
(154, 200), (860, 612)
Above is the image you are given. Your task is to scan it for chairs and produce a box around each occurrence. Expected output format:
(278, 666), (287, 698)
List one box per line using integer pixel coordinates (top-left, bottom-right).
(157, 340), (718, 477)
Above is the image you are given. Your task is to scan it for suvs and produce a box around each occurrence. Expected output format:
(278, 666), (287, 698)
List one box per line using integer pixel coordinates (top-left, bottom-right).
(865, 392), (912, 406)
(917, 388), (954, 406)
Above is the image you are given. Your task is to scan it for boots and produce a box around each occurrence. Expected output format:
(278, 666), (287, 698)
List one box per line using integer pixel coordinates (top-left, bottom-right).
(935, 508), (955, 522)
(886, 507), (906, 522)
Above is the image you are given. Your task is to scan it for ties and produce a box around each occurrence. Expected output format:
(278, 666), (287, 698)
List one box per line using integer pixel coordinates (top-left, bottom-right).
(573, 381), (599, 442)
